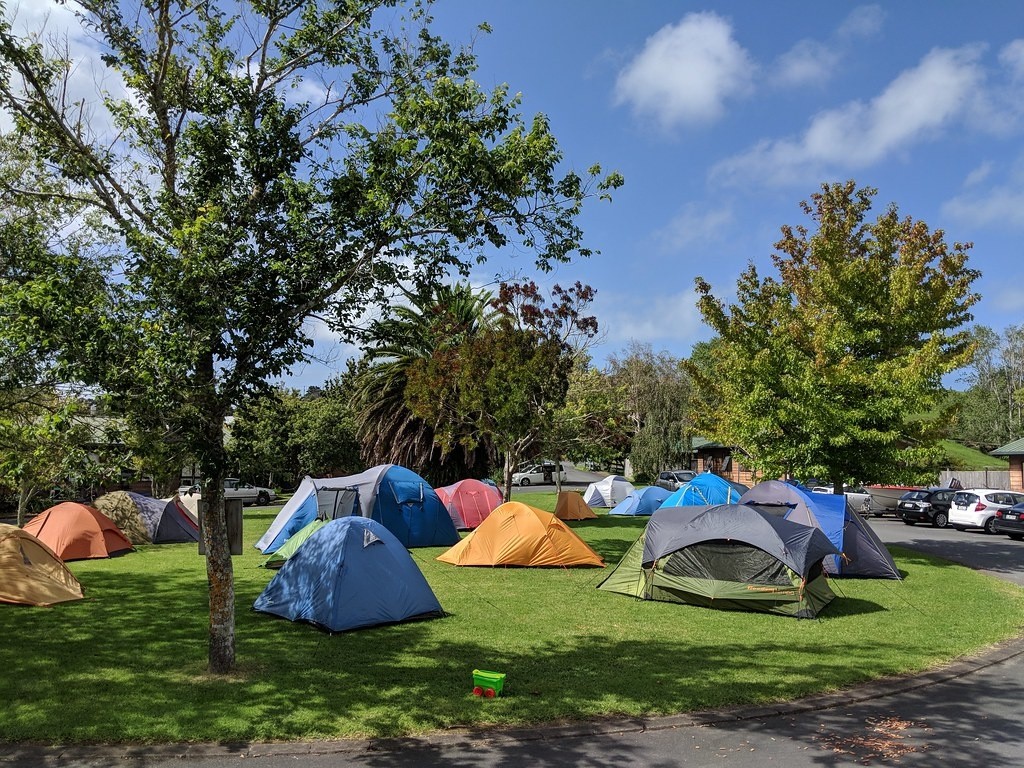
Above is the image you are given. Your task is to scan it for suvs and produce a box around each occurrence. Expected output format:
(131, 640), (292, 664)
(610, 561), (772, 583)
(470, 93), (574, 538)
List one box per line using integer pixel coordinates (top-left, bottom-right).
(897, 487), (961, 528)
(947, 489), (1024, 535)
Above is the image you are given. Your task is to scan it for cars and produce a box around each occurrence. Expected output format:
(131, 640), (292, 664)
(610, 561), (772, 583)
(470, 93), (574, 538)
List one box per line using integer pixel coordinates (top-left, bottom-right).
(811, 481), (885, 517)
(178, 477), (277, 506)
(655, 470), (698, 492)
(991, 502), (1024, 541)
(511, 462), (568, 487)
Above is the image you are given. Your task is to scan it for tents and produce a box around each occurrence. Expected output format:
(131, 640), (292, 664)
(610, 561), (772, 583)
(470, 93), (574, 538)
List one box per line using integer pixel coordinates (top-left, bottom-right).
(0, 491), (199, 605)
(251, 465), (903, 636)
